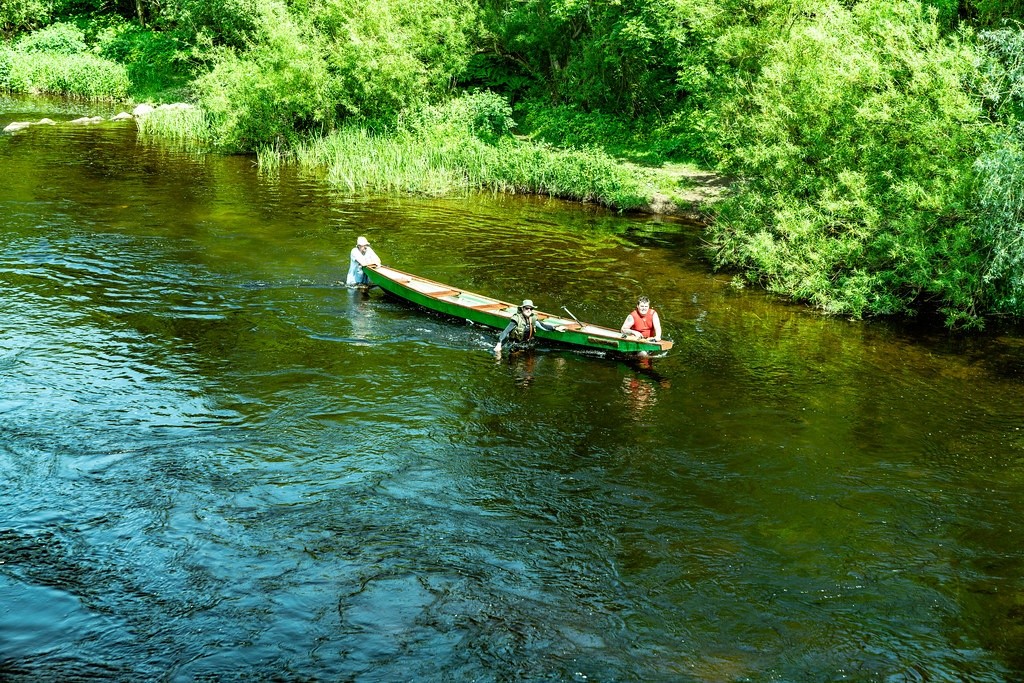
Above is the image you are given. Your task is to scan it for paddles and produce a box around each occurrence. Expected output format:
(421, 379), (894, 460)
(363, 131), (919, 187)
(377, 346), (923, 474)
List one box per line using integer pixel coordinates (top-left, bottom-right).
(560, 305), (589, 330)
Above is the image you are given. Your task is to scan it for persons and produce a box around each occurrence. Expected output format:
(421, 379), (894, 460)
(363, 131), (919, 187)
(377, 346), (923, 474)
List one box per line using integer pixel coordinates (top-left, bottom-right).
(621, 296), (662, 356)
(346, 236), (381, 286)
(493, 300), (568, 352)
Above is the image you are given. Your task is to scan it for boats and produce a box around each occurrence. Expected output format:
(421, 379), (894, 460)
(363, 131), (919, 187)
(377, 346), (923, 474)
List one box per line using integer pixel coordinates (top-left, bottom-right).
(361, 262), (674, 353)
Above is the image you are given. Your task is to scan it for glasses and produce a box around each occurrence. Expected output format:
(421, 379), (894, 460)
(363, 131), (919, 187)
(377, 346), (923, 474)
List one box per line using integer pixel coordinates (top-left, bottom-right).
(523, 306), (533, 310)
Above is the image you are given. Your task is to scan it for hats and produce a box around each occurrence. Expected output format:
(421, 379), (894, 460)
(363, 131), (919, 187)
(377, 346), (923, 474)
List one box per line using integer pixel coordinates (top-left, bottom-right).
(517, 300), (538, 311)
(356, 237), (370, 246)
